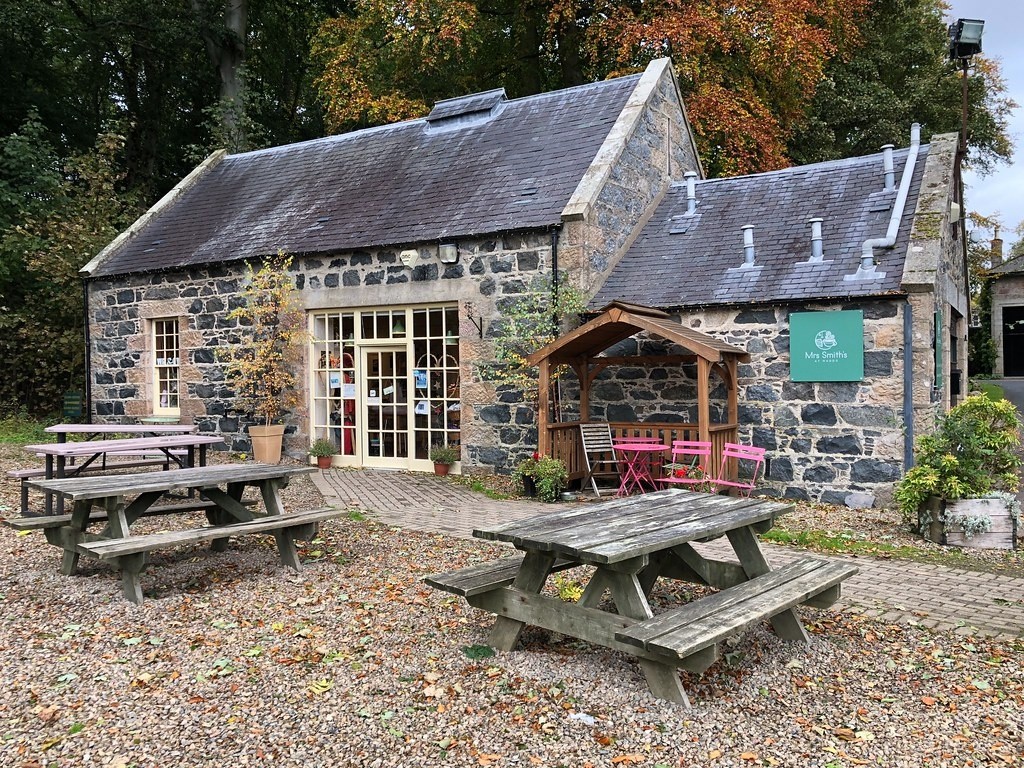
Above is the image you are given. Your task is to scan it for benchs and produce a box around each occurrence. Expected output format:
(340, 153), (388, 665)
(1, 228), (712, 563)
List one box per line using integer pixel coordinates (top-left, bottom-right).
(75, 507), (350, 576)
(424, 553), (590, 616)
(4, 457), (177, 518)
(613, 554), (859, 674)
(36, 448), (188, 466)
(1, 498), (258, 549)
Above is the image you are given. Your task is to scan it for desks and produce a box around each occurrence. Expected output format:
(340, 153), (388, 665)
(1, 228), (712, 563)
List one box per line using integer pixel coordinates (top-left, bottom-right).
(471, 488), (811, 712)
(44, 423), (200, 467)
(612, 443), (670, 497)
(611, 435), (663, 493)
(24, 461), (318, 607)
(23, 434), (225, 517)
(140, 416), (181, 460)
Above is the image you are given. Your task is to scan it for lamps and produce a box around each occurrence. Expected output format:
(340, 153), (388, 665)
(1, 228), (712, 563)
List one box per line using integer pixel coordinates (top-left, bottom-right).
(393, 318), (406, 335)
(441, 330), (459, 345)
(344, 333), (354, 347)
(438, 242), (460, 265)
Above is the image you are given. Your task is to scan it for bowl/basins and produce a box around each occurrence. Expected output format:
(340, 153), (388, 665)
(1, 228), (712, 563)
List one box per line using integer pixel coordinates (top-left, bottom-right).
(560, 492), (581, 501)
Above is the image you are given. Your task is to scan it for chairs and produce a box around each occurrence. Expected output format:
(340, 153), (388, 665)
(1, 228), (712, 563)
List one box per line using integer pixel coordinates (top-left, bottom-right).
(579, 422), (629, 497)
(370, 418), (388, 457)
(710, 442), (766, 497)
(654, 441), (712, 493)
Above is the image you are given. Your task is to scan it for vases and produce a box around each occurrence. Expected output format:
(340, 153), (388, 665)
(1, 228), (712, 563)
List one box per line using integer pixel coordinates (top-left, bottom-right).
(522, 474), (539, 497)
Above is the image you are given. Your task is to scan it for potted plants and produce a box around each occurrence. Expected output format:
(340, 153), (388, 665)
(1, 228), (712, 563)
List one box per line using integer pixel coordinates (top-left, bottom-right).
(213, 249), (325, 465)
(892, 389), (1024, 551)
(309, 437), (340, 470)
(429, 443), (459, 476)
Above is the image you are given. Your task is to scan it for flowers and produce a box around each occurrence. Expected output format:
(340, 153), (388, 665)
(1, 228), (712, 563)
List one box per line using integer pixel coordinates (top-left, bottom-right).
(509, 451), (571, 503)
(668, 464), (719, 495)
